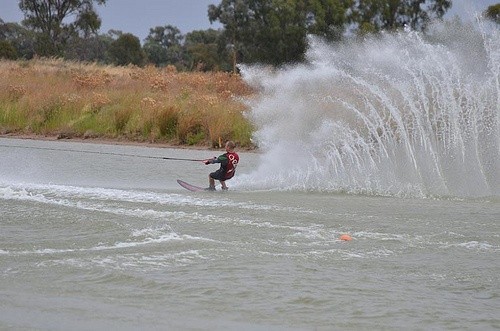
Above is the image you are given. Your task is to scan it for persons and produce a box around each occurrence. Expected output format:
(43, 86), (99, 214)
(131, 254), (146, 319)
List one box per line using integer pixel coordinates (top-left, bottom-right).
(205, 140), (240, 192)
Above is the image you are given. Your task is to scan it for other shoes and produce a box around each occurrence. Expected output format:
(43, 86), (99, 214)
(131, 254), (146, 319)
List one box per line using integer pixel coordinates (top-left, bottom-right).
(204, 187), (216, 191)
(221, 186), (228, 191)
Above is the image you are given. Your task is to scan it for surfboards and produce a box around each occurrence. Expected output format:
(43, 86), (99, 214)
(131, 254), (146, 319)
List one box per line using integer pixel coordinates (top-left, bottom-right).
(177, 178), (206, 192)
